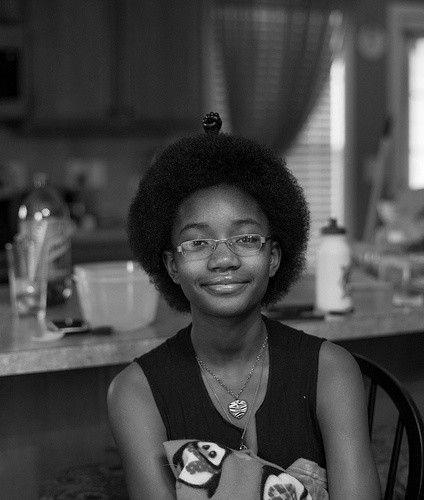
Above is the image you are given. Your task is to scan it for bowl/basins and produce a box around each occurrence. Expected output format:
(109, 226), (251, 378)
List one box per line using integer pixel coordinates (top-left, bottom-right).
(72, 260), (160, 333)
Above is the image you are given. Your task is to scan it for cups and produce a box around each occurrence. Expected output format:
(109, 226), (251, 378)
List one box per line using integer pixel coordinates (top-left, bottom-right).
(6, 242), (50, 318)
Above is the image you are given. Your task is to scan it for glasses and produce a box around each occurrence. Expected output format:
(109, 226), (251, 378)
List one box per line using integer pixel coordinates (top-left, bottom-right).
(166, 232), (276, 261)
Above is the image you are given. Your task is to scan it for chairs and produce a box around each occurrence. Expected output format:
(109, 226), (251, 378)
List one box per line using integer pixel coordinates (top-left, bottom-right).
(349, 351), (424, 500)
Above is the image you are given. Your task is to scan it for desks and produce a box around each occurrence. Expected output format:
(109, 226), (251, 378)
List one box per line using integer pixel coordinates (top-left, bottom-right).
(0, 269), (424, 500)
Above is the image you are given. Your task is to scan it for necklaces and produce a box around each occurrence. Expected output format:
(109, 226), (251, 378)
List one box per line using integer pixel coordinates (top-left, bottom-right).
(192, 342), (267, 452)
(187, 335), (269, 420)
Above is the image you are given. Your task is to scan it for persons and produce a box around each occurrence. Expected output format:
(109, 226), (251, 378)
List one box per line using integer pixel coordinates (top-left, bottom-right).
(106, 133), (382, 500)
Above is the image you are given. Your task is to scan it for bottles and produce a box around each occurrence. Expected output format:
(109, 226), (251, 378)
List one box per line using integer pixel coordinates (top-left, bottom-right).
(18, 173), (72, 307)
(315, 219), (354, 315)
